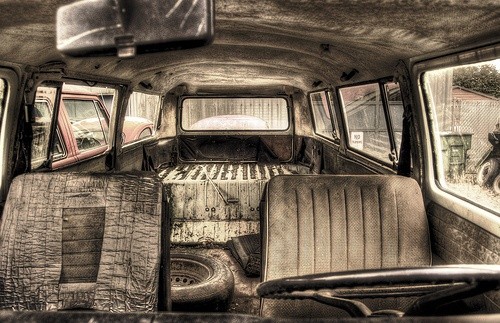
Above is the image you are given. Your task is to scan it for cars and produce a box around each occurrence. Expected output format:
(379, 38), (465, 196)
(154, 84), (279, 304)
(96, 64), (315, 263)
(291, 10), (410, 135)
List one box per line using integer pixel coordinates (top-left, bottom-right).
(0, 90), (152, 171)
